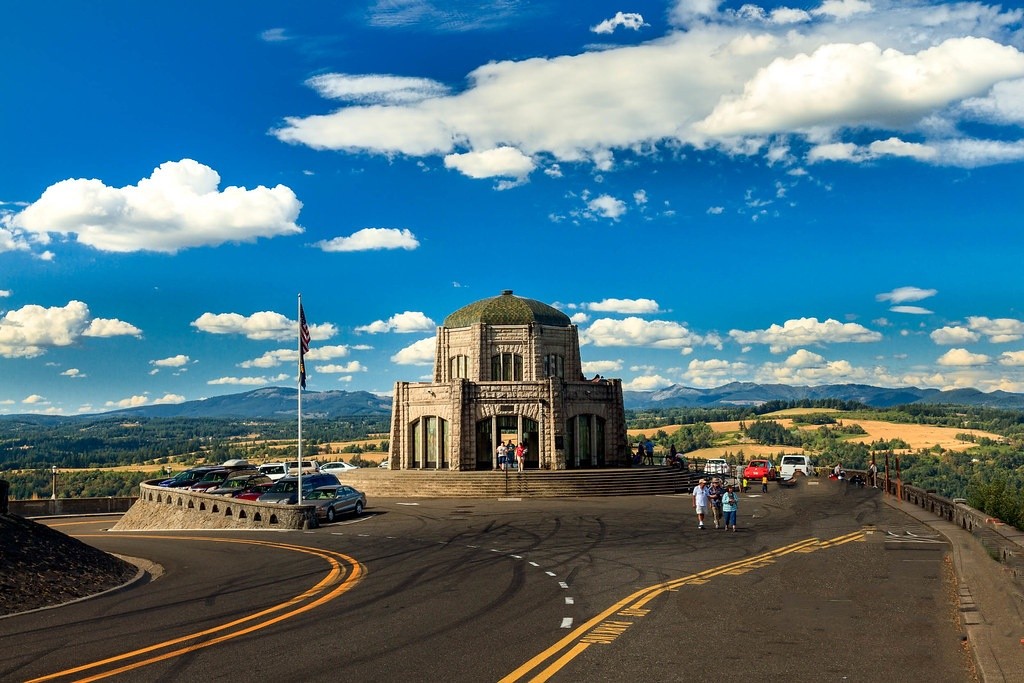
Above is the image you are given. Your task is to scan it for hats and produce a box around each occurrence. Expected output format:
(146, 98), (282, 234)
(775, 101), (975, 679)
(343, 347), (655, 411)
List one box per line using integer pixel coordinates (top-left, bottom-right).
(710, 478), (721, 483)
(699, 478), (707, 484)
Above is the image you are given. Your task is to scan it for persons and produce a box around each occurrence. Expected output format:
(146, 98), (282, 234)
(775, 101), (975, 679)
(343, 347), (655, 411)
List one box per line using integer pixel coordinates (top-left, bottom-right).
(662, 444), (684, 470)
(693, 477), (738, 532)
(736, 462), (751, 493)
(762, 474), (768, 493)
(850, 472), (866, 488)
(495, 440), (526, 472)
(816, 464), (821, 476)
(770, 466), (780, 479)
(831, 462), (849, 483)
(867, 461), (877, 486)
(808, 463), (816, 476)
(632, 439), (654, 465)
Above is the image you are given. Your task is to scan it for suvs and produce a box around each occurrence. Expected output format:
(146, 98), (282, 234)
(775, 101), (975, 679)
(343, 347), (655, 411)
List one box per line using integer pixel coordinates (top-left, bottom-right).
(780, 455), (815, 481)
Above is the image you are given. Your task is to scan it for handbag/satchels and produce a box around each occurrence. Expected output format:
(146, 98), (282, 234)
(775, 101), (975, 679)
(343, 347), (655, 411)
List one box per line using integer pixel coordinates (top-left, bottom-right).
(867, 469), (874, 477)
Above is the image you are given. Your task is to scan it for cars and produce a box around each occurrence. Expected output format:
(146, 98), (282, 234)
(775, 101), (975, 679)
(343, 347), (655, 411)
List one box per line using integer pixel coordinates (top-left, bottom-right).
(744, 460), (777, 481)
(703, 459), (730, 474)
(378, 461), (388, 469)
(158, 460), (367, 524)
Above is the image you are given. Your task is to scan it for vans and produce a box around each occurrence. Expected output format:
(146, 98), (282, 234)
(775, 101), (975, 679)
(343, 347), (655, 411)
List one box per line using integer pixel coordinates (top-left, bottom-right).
(283, 461), (320, 476)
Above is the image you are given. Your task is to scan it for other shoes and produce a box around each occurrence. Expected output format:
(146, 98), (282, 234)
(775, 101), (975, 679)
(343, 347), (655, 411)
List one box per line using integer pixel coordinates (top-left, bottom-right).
(510, 464), (514, 468)
(763, 491), (767, 493)
(518, 469), (524, 473)
(713, 522), (720, 529)
(732, 526), (738, 533)
(697, 522), (704, 530)
(501, 468), (504, 472)
(724, 525), (729, 532)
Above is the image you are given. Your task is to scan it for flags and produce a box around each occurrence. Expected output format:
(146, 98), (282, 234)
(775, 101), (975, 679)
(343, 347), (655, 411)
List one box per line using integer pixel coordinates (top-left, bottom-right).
(299, 303), (311, 391)
